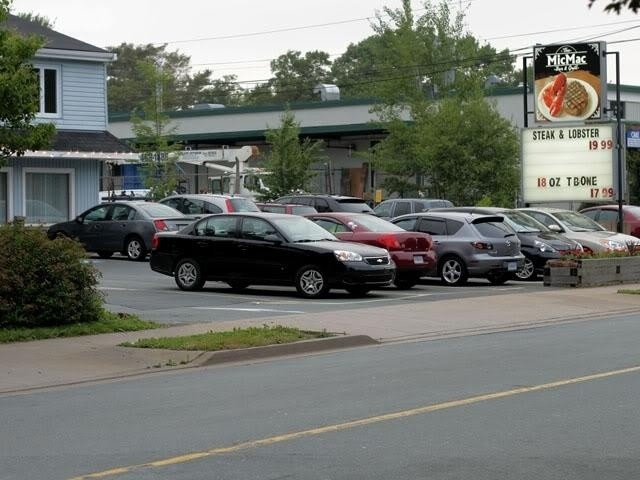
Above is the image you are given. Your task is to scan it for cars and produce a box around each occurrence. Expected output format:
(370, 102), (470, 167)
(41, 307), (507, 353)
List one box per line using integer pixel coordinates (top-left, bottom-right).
(387, 211), (526, 286)
(576, 205), (640, 238)
(1, 197), (67, 223)
(515, 206), (640, 259)
(159, 195), (259, 217)
(48, 202), (197, 263)
(259, 202), (317, 217)
(150, 212), (397, 299)
(426, 207), (584, 281)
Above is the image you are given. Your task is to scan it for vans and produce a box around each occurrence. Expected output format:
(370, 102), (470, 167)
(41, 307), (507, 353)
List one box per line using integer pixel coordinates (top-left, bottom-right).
(374, 198), (454, 221)
(100, 188), (178, 203)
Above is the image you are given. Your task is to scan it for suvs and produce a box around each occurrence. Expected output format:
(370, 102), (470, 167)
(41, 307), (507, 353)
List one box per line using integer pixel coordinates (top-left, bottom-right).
(274, 192), (377, 217)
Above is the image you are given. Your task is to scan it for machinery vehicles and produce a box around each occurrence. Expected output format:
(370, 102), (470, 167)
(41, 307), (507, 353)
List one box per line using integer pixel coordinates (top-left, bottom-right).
(105, 140), (304, 202)
